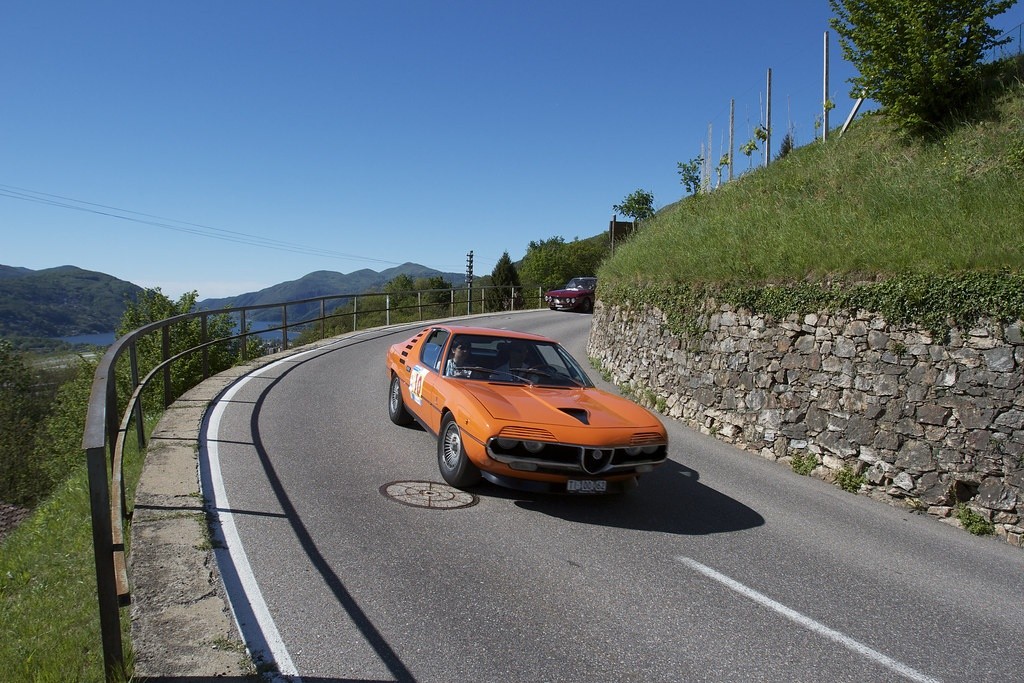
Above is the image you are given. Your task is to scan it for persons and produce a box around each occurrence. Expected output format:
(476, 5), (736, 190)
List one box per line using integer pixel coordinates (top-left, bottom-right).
(436, 337), (483, 379)
(490, 343), (542, 384)
(572, 279), (595, 290)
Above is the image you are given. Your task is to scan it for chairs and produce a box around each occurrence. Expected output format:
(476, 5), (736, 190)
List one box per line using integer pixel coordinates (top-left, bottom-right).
(492, 349), (509, 370)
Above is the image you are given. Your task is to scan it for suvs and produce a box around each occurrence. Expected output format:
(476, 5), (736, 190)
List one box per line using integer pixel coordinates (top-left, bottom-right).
(544, 276), (598, 312)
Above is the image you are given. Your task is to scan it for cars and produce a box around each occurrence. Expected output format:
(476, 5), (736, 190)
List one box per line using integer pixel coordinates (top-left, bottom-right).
(389, 325), (668, 498)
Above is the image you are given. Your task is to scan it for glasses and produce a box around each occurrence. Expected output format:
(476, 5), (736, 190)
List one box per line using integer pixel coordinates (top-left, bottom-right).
(453, 346), (471, 350)
(512, 349), (528, 354)
